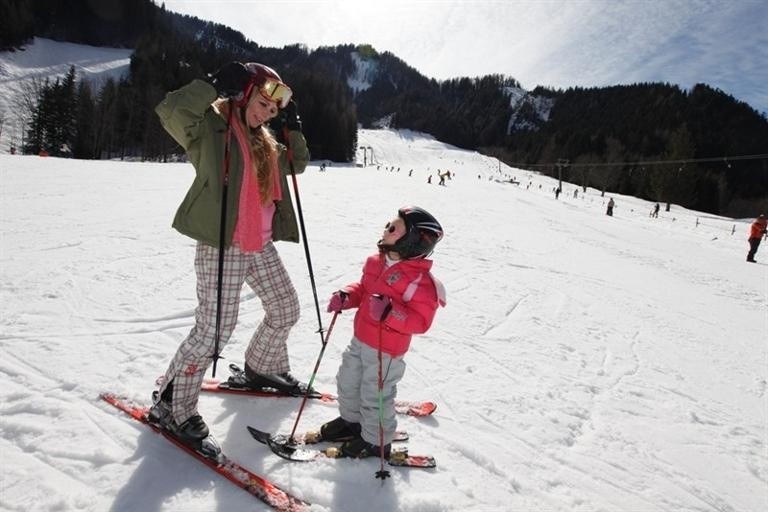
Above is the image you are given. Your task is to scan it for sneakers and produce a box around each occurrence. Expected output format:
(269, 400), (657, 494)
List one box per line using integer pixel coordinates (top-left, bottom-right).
(156, 389), (207, 439)
(243, 361), (297, 386)
(320, 416), (361, 442)
(342, 438), (392, 458)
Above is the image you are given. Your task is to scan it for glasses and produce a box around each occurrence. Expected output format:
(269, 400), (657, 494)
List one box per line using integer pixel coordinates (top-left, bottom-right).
(384, 222), (401, 237)
(250, 71), (293, 110)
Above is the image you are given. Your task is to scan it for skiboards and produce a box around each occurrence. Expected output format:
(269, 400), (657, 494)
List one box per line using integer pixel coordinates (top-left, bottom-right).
(156, 376), (435, 423)
(246, 420), (435, 474)
(101, 390), (335, 511)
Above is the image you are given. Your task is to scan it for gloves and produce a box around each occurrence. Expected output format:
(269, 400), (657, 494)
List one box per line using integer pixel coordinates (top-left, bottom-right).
(264, 98), (297, 128)
(211, 60), (248, 93)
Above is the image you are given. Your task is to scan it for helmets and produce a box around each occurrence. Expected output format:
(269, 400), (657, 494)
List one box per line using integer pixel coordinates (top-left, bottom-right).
(395, 206), (443, 260)
(230, 63), (283, 106)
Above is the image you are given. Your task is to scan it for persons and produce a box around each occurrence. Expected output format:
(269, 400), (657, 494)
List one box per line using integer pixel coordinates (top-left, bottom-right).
(319, 163), (327, 171)
(606, 197), (614, 216)
(324, 206), (448, 460)
(573, 189), (579, 199)
(746, 210), (768, 264)
(147, 59), (310, 442)
(427, 175), (432, 183)
(447, 170), (451, 180)
(652, 202), (659, 218)
(555, 187), (560, 199)
(438, 172), (449, 185)
(59, 136), (73, 153)
